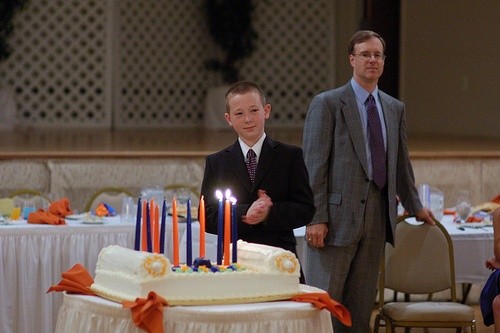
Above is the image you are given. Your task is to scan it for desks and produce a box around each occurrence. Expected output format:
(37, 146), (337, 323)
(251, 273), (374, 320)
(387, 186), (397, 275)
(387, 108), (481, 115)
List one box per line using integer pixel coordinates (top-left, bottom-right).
(292, 209), (496, 291)
(0, 212), (219, 333)
(53, 284), (334, 333)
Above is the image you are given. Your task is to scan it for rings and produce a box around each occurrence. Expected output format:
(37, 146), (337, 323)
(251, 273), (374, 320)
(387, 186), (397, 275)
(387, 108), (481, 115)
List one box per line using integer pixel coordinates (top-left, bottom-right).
(308, 238), (313, 241)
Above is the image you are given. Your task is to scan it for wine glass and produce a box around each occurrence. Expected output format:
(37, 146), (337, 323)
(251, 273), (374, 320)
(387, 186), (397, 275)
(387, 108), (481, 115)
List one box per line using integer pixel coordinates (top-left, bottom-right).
(455, 203), (470, 226)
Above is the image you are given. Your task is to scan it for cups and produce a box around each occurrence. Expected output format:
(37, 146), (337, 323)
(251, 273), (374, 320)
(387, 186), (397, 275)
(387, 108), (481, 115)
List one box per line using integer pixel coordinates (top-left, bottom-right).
(120, 185), (190, 224)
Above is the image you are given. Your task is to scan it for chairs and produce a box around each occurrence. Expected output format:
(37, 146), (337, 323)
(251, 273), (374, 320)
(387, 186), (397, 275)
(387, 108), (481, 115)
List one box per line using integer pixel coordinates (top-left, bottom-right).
(373, 216), (476, 333)
(10, 183), (200, 210)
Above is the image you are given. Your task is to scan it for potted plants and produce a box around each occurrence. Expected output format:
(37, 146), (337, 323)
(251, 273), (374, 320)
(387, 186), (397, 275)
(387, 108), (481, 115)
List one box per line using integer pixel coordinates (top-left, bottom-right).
(198, 0), (257, 132)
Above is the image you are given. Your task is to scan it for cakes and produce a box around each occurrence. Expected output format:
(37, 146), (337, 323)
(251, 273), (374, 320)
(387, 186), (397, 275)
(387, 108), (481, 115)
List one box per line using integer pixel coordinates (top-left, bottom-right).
(89, 238), (301, 301)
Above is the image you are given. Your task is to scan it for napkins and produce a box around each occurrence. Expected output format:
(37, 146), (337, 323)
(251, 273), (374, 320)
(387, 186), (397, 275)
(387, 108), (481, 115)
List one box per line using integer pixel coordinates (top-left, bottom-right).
(26, 197), (117, 224)
(444, 209), (456, 215)
(466, 216), (482, 223)
(120, 291), (169, 333)
(290, 292), (352, 328)
(46, 263), (98, 297)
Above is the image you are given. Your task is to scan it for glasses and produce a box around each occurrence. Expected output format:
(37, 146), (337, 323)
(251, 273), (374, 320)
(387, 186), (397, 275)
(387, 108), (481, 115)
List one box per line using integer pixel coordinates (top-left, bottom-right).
(353, 52), (388, 62)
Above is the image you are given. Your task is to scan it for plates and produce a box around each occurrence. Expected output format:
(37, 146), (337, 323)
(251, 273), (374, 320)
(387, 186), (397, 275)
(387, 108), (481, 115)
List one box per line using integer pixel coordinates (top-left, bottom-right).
(0, 215), (106, 225)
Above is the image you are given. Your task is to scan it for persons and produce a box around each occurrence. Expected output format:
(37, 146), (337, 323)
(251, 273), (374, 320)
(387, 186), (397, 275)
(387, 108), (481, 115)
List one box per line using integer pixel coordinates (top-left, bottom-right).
(486, 207), (500, 333)
(198, 81), (315, 284)
(304, 31), (436, 333)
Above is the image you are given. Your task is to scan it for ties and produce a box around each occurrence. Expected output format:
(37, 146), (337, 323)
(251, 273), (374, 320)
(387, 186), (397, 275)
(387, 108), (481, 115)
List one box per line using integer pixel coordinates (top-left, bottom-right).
(363, 94), (387, 190)
(246, 149), (258, 183)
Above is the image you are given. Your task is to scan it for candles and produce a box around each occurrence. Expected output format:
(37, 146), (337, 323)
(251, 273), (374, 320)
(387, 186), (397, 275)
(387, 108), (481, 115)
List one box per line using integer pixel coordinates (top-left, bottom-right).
(135, 189), (238, 268)
